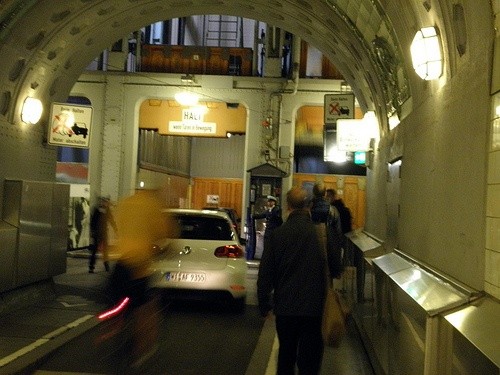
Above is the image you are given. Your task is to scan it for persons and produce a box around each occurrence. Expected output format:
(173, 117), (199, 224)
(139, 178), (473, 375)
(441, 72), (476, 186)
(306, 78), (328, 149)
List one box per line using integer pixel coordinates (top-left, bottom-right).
(87, 195), (119, 272)
(328, 187), (352, 240)
(309, 184), (341, 290)
(255, 191), (335, 375)
(253, 195), (283, 249)
(323, 189), (341, 278)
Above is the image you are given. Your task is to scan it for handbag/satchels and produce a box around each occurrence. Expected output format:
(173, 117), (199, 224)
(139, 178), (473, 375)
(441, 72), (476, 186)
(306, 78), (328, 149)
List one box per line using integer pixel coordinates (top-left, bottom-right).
(321, 289), (345, 347)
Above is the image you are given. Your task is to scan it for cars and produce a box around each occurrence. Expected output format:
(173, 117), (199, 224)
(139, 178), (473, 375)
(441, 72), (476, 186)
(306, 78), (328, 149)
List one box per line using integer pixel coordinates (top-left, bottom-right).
(133, 208), (247, 309)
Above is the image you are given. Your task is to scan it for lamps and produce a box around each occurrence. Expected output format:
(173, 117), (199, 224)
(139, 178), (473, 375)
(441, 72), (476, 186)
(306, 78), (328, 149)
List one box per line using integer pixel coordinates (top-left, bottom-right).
(409, 27), (444, 80)
(20, 96), (43, 124)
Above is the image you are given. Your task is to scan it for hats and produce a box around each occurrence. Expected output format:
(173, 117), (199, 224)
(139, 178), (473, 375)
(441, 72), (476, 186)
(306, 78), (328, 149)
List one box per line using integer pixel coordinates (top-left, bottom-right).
(267, 195), (277, 201)
